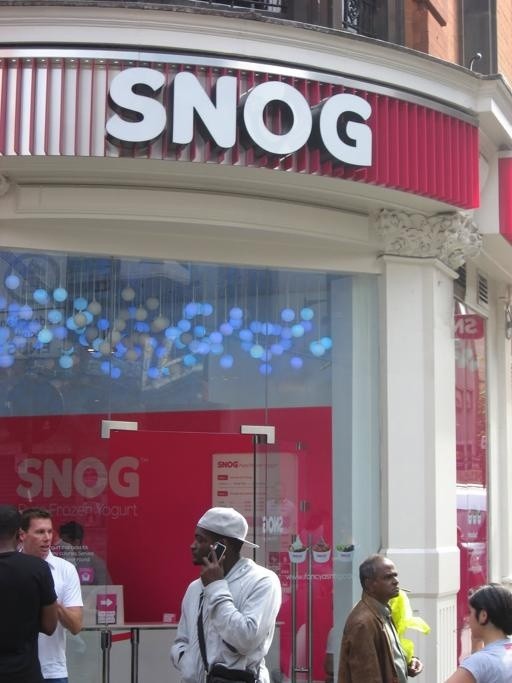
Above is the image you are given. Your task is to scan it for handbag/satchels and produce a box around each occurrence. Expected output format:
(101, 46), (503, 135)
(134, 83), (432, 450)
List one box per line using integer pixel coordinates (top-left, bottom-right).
(202, 662), (261, 683)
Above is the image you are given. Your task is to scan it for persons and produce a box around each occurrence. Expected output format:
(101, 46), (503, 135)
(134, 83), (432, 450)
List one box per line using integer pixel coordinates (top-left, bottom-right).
(336, 552), (424, 683)
(443, 582), (512, 682)
(0, 503), (58, 683)
(170, 505), (283, 683)
(18, 508), (84, 682)
(61, 520), (112, 584)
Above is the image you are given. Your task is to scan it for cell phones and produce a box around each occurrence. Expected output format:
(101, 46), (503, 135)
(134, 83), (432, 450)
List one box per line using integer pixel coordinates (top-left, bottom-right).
(207, 541), (227, 563)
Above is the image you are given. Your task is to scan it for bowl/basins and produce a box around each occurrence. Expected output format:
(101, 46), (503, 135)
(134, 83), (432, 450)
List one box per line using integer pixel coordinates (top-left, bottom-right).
(336, 544), (356, 563)
(288, 545), (307, 562)
(312, 546), (331, 563)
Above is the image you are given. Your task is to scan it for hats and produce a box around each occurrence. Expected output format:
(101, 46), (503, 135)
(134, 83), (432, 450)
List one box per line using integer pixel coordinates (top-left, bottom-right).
(195, 505), (262, 550)
